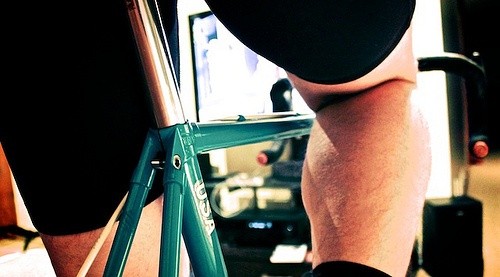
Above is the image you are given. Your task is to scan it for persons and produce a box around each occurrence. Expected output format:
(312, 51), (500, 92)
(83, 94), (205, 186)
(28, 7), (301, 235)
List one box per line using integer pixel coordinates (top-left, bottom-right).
(1, 1), (433, 277)
